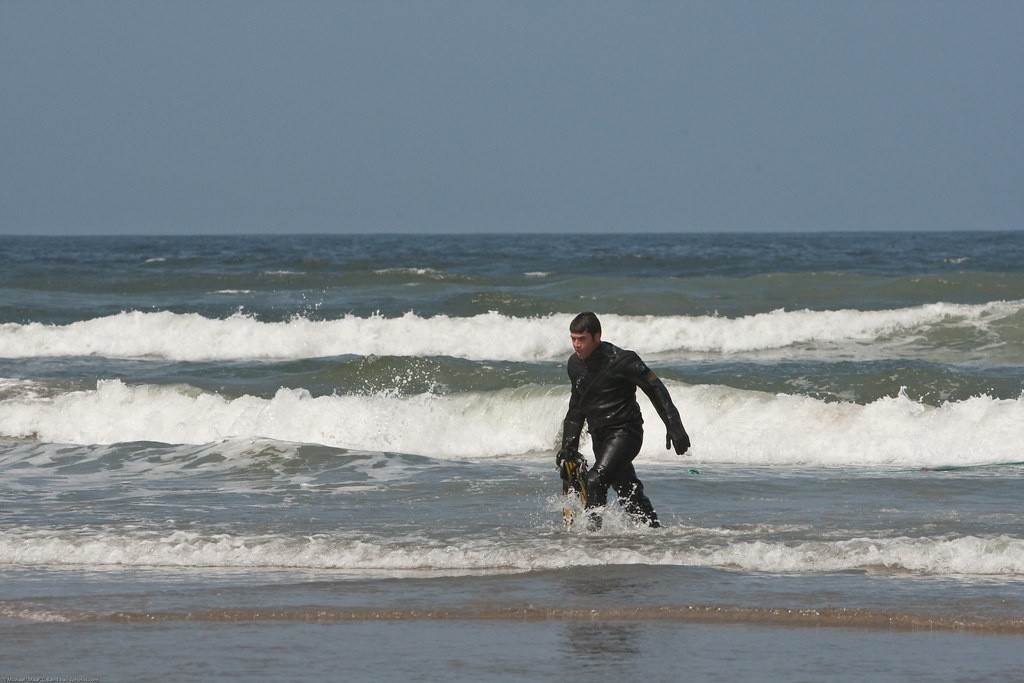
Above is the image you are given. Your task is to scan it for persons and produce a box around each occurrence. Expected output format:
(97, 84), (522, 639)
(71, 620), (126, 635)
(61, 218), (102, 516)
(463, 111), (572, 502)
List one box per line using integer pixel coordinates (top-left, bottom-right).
(556, 312), (691, 528)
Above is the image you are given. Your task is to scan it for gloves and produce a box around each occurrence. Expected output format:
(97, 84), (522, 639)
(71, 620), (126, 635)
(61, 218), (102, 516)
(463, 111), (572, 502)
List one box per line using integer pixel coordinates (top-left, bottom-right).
(665, 417), (690, 455)
(555, 421), (582, 465)
